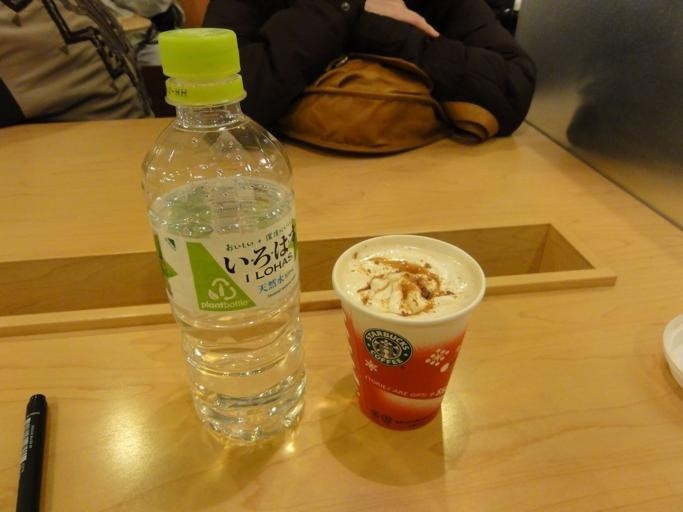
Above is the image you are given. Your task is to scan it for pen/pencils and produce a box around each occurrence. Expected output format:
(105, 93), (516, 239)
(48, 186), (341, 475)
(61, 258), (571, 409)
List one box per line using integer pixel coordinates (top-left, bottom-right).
(15, 394), (47, 512)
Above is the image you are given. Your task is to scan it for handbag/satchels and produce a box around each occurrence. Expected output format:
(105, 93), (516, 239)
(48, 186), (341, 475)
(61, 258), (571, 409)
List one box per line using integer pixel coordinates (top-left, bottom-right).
(283, 53), (449, 154)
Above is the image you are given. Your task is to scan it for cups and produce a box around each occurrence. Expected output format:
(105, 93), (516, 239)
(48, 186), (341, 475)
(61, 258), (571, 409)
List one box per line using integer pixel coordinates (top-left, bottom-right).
(331, 234), (486, 430)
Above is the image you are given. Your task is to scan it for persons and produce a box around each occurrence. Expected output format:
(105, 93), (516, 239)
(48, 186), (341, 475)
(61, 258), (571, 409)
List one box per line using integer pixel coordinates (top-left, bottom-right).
(202, 0), (537, 137)
(0, 0), (209, 130)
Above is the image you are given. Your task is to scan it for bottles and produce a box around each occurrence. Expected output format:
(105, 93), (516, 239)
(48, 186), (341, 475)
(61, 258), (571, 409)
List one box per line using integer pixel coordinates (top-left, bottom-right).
(141, 28), (306, 447)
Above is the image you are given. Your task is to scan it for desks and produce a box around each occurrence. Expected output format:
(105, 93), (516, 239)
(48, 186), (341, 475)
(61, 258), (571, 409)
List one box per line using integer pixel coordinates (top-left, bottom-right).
(524, 83), (682, 231)
(0, 117), (682, 512)
